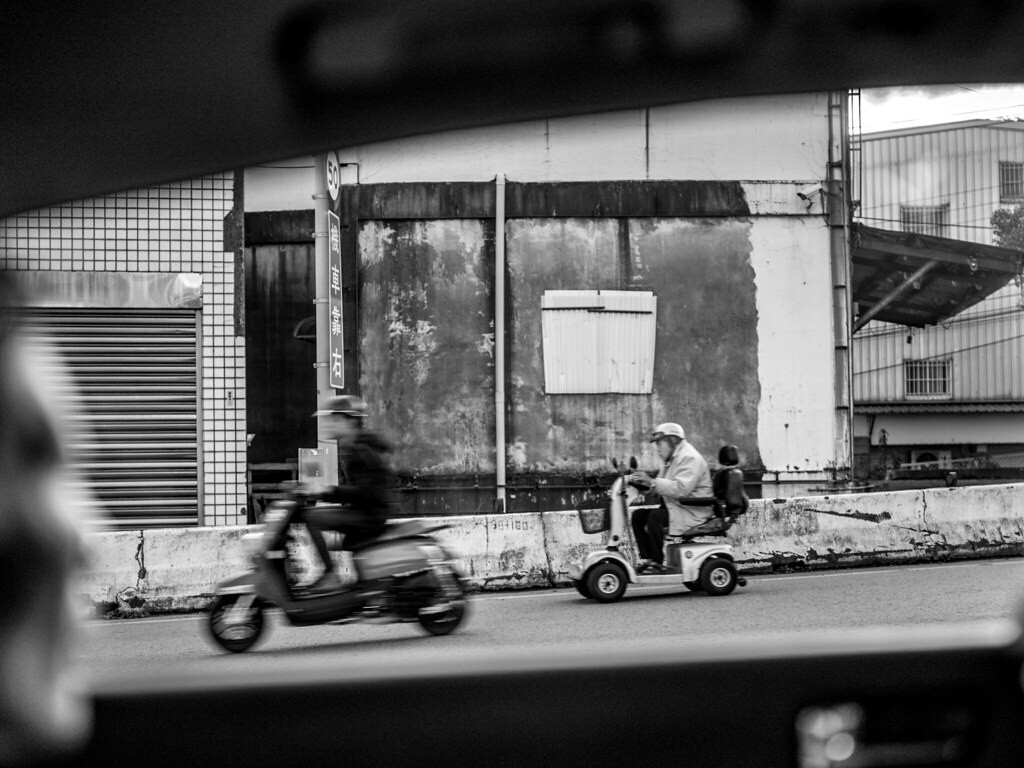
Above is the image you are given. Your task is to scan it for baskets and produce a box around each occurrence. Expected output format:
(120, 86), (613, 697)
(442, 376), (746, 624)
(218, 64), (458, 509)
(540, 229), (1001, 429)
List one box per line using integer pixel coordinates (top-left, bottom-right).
(575, 498), (610, 534)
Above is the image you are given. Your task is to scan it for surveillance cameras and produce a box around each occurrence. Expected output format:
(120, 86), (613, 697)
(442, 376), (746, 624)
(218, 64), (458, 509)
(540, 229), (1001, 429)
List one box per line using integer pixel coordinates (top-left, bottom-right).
(797, 184), (823, 200)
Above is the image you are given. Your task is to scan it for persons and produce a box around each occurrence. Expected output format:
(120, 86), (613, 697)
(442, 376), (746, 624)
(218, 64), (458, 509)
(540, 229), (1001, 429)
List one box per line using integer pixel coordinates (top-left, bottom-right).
(629, 422), (716, 574)
(302, 397), (395, 598)
(1, 270), (128, 753)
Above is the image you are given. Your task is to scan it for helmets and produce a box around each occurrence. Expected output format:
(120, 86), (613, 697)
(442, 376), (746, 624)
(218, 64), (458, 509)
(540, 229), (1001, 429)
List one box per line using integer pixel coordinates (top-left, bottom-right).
(314, 394), (369, 417)
(650, 423), (685, 441)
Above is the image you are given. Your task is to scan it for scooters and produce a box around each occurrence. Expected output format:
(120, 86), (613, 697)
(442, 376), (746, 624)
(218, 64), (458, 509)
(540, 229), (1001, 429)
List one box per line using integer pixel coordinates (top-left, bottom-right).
(571, 444), (751, 605)
(203, 476), (466, 654)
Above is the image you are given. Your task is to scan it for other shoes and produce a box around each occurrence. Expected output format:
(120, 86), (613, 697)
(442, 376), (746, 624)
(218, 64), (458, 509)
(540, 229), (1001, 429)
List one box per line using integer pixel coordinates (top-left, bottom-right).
(637, 564), (649, 573)
(642, 565), (666, 574)
(306, 573), (345, 591)
